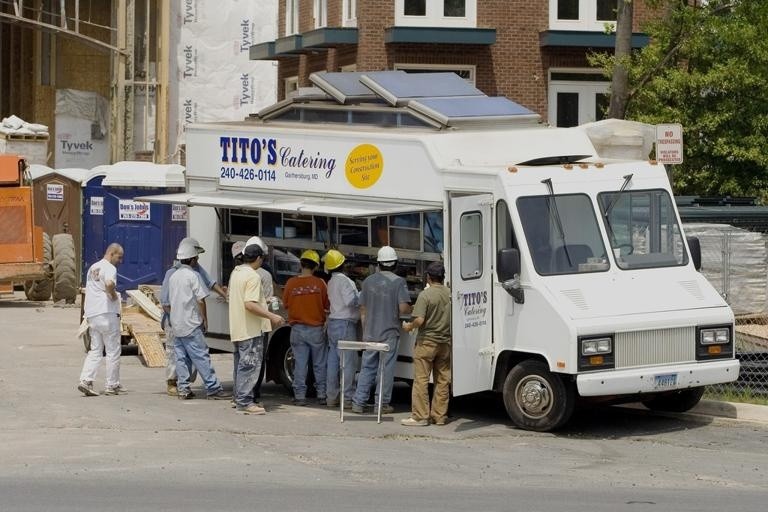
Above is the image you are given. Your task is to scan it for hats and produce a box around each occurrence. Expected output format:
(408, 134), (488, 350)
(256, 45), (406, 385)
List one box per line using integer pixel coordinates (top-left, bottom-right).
(423, 260), (446, 277)
(244, 244), (267, 262)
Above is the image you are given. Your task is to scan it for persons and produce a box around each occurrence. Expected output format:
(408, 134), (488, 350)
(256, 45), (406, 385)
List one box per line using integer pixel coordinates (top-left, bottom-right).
(283, 249), (330, 406)
(159, 237), (227, 397)
(168, 243), (234, 400)
(401, 261), (452, 426)
(322, 249), (360, 409)
(352, 245), (413, 415)
(241, 234), (274, 402)
(76, 242), (129, 395)
(229, 244), (287, 416)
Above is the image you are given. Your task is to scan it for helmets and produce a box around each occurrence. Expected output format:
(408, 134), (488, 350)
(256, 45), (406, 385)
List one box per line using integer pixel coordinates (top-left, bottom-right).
(375, 245), (399, 267)
(240, 234), (270, 258)
(178, 236), (206, 253)
(176, 245), (199, 261)
(299, 249), (321, 268)
(322, 247), (347, 272)
(231, 240), (248, 260)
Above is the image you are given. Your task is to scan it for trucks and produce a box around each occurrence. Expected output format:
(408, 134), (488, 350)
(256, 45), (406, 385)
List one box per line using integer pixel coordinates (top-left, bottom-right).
(129, 69), (739, 432)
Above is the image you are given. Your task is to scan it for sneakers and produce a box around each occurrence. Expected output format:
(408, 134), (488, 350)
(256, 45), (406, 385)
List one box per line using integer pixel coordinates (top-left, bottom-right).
(316, 397), (328, 406)
(326, 399), (341, 408)
(429, 416), (446, 426)
(372, 404), (394, 415)
(342, 399), (353, 410)
(76, 379), (101, 397)
(290, 398), (308, 406)
(351, 403), (365, 415)
(400, 416), (429, 427)
(176, 386), (206, 400)
(103, 384), (130, 397)
(204, 389), (234, 400)
(230, 397), (268, 416)
(165, 379), (180, 397)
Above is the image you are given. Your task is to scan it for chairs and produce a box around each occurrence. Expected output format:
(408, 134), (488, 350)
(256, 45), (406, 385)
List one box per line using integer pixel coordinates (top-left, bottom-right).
(551, 245), (593, 272)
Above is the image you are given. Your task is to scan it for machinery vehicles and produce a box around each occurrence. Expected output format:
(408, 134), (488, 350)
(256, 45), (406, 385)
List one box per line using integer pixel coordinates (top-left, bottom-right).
(0, 153), (78, 304)
